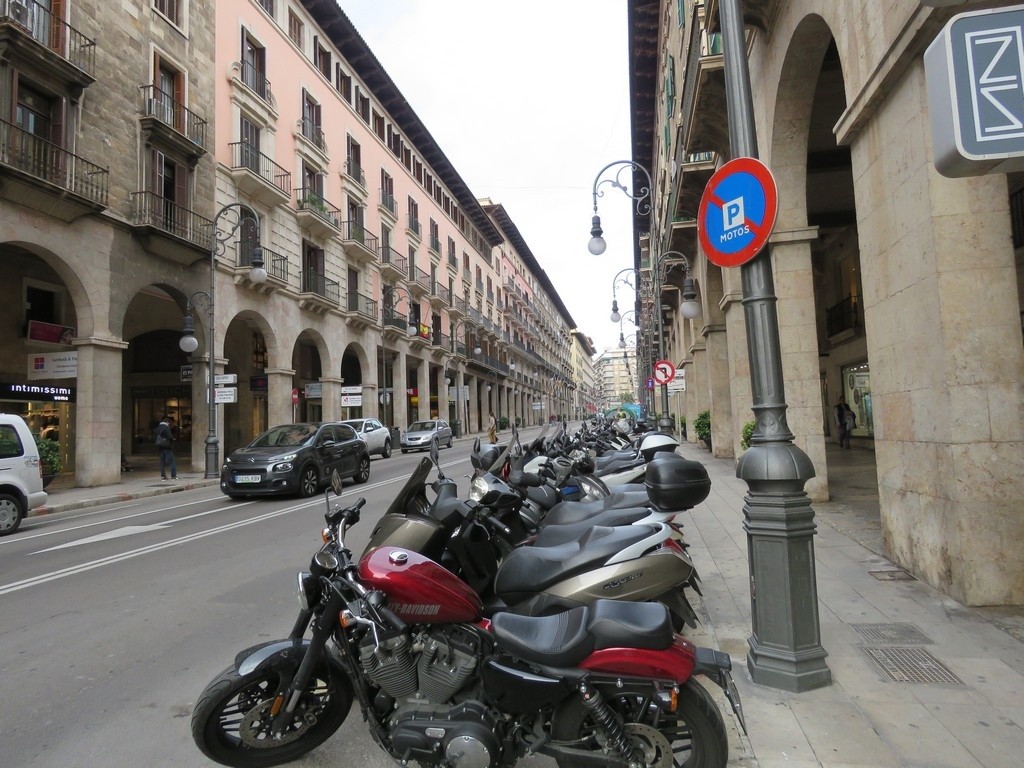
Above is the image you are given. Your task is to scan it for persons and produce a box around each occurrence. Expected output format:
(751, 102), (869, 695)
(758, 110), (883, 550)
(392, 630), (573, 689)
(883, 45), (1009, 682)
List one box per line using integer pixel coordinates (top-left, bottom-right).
(487, 412), (498, 445)
(154, 418), (181, 482)
(833, 396), (856, 449)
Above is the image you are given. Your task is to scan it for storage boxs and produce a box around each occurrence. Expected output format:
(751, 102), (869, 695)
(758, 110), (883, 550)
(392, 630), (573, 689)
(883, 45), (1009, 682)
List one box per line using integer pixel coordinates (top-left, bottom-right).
(639, 423), (712, 509)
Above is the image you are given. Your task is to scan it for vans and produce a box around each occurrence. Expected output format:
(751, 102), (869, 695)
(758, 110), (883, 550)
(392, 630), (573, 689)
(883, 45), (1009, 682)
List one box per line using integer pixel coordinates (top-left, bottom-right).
(0, 412), (49, 538)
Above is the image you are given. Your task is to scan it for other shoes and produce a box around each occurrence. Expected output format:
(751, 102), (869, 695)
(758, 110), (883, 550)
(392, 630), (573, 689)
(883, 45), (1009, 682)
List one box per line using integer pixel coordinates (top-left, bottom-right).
(161, 477), (169, 481)
(171, 476), (181, 482)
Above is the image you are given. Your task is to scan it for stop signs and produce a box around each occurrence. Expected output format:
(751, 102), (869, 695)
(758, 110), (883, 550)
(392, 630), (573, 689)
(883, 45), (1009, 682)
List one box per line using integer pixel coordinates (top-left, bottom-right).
(291, 388), (299, 404)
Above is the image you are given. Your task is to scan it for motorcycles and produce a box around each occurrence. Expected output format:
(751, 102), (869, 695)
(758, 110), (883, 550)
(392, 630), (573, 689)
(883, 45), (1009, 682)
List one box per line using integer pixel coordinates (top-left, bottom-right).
(187, 470), (750, 768)
(333, 411), (713, 642)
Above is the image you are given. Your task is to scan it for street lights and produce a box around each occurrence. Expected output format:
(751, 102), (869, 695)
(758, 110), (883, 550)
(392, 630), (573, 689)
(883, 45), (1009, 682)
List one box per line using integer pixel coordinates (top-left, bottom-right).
(455, 320), (482, 439)
(381, 285), (418, 430)
(587, 160), (676, 441)
(178, 200), (269, 480)
(609, 250), (701, 431)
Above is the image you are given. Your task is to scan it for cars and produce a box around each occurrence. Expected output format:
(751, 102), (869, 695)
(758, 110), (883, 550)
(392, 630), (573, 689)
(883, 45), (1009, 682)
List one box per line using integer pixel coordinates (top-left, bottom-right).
(334, 417), (392, 460)
(400, 419), (454, 454)
(219, 421), (371, 500)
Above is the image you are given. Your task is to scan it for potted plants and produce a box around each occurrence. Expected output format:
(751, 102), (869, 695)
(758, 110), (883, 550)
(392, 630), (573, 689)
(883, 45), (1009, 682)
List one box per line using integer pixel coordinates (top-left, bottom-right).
(451, 419), (461, 435)
(499, 416), (509, 429)
(680, 417), (686, 437)
(695, 412), (711, 451)
(303, 195), (332, 221)
(0, 430), (63, 488)
(515, 417), (520, 427)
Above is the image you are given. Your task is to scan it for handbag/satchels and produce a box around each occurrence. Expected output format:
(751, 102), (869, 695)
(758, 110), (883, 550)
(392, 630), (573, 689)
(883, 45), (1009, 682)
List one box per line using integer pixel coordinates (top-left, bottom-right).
(156, 430), (170, 448)
